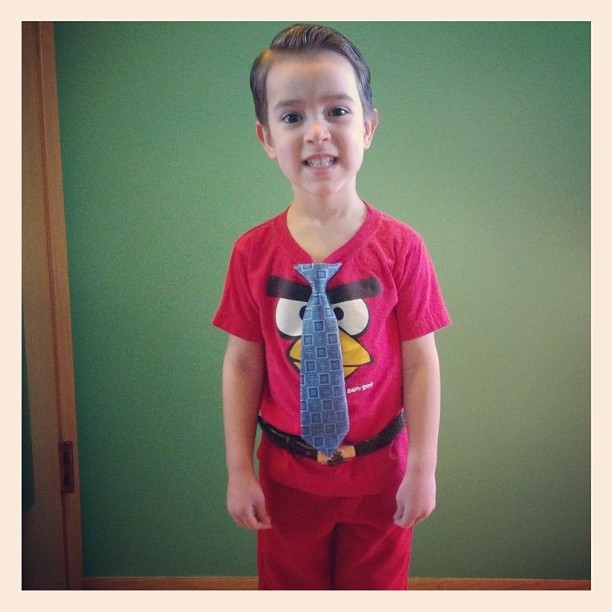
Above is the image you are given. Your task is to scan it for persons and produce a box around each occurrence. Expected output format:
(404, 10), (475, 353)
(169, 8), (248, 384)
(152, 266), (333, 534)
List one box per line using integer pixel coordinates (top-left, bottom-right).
(210, 22), (453, 590)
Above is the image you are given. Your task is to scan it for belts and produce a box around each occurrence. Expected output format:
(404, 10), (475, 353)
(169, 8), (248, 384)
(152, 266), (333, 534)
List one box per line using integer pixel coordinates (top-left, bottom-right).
(257, 410), (404, 465)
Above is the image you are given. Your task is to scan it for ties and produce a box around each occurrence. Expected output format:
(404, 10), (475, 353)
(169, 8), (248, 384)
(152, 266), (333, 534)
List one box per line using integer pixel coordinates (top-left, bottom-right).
(294, 263), (350, 457)
